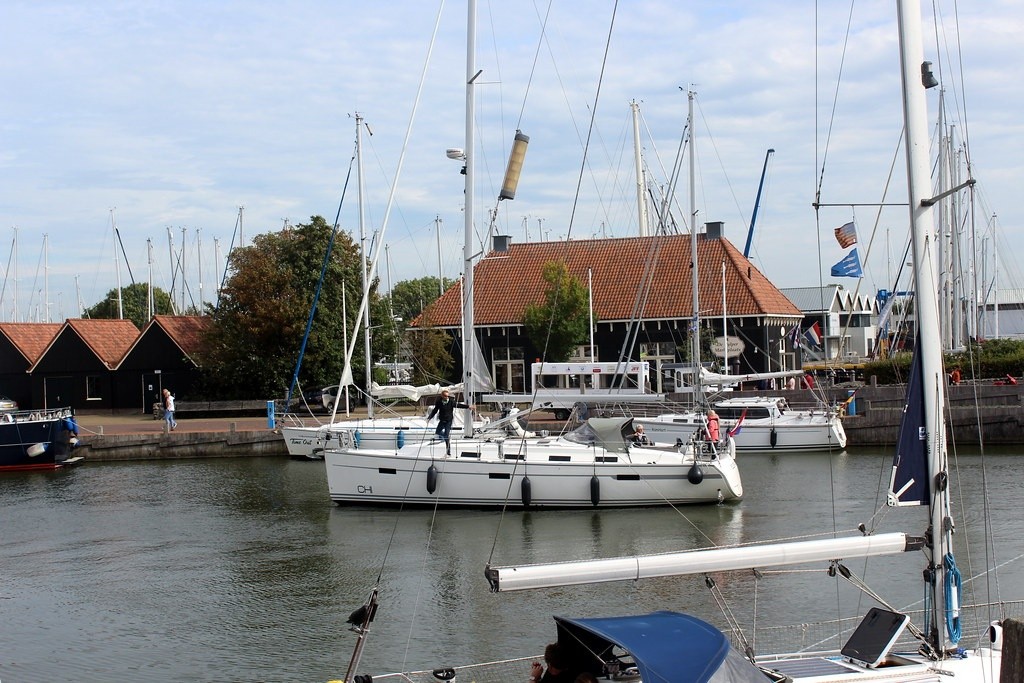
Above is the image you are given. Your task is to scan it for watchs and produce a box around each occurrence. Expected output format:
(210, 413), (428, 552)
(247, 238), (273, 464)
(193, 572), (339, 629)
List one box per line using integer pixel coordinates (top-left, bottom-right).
(529, 676), (537, 680)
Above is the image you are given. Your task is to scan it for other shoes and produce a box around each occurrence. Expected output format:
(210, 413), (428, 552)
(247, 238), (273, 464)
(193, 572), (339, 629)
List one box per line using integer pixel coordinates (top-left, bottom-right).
(172, 423), (177, 430)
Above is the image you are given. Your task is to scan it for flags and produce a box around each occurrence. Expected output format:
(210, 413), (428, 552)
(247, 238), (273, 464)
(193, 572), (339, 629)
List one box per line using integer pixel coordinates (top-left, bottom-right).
(788, 323), (803, 350)
(803, 324), (822, 345)
(831, 249), (861, 277)
(839, 387), (859, 408)
(730, 407), (747, 437)
(836, 223), (856, 247)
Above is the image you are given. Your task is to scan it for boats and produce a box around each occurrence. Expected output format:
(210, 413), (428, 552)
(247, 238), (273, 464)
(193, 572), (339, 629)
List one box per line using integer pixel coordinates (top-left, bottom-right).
(0, 407), (85, 473)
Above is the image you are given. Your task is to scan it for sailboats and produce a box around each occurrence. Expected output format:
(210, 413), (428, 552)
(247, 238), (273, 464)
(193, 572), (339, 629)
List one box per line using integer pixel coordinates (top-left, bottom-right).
(614, 83), (848, 457)
(319, 0), (746, 508)
(264, 110), (540, 458)
(278, 0), (1024, 682)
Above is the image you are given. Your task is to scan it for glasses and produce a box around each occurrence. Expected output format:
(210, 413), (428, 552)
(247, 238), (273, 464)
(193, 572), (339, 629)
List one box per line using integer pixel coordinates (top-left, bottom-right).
(444, 395), (448, 397)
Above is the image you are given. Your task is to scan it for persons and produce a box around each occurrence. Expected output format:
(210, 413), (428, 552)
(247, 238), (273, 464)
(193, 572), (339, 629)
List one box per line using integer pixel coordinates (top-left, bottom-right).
(162, 389), (177, 432)
(767, 371), (813, 392)
(702, 411), (720, 460)
(527, 643), (598, 683)
(625, 424), (651, 446)
(948, 335), (1016, 384)
(428, 390), (476, 456)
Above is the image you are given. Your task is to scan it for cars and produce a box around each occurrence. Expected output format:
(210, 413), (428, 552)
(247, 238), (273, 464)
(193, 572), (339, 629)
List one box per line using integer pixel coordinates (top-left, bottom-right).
(0, 394), (21, 417)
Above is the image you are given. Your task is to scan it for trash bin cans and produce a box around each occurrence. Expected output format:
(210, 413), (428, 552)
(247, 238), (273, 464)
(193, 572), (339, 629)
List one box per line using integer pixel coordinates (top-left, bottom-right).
(848, 390), (856, 414)
(266, 400), (275, 428)
